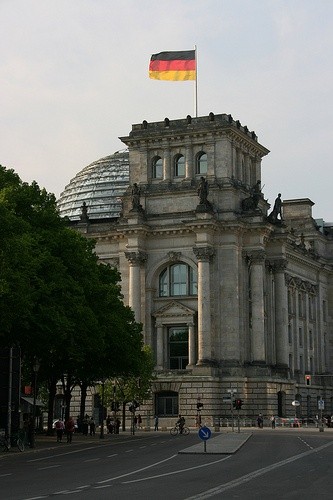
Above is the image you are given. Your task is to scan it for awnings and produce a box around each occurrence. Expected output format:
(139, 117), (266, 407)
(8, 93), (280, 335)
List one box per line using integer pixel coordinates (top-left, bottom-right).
(21, 397), (44, 406)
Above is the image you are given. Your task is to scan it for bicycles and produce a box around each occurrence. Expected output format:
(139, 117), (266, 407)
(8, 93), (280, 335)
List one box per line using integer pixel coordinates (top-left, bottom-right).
(170, 423), (190, 437)
(13, 427), (27, 452)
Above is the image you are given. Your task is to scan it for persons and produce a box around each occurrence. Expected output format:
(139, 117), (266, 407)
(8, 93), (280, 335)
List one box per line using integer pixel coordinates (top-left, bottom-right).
(270, 415), (276, 430)
(56, 417), (75, 445)
(196, 177), (209, 204)
(153, 415), (159, 431)
(77, 414), (97, 436)
(130, 183), (144, 212)
(105, 415), (121, 434)
(134, 414), (142, 430)
(177, 415), (186, 434)
(318, 416), (333, 428)
(280, 416), (304, 427)
(290, 227), (295, 235)
(79, 202), (90, 223)
(300, 233), (305, 246)
(272, 193), (286, 222)
(257, 413), (264, 427)
(251, 179), (263, 208)
(23, 432), (35, 449)
(195, 415), (201, 427)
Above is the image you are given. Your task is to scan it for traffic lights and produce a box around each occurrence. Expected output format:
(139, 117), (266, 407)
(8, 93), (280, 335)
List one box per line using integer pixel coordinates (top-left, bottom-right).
(233, 400), (236, 408)
(94, 394), (101, 408)
(239, 400), (243, 409)
(197, 402), (203, 408)
(111, 402), (115, 411)
(116, 402), (120, 412)
(305, 375), (311, 386)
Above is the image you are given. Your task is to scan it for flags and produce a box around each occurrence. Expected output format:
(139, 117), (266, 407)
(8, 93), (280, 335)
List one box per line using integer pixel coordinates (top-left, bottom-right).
(149, 50), (196, 81)
